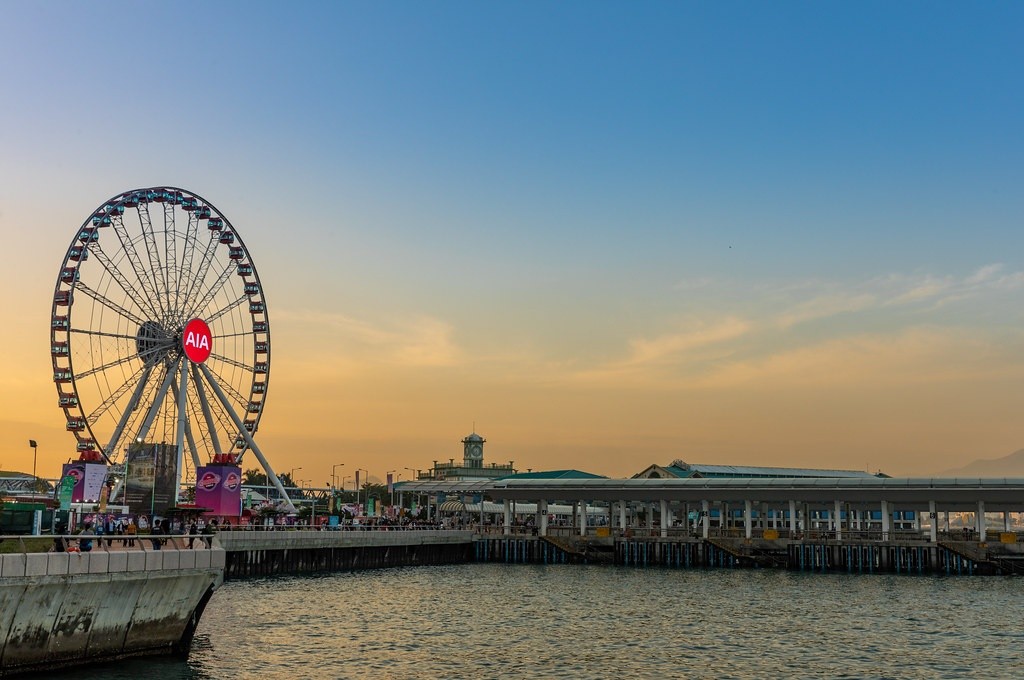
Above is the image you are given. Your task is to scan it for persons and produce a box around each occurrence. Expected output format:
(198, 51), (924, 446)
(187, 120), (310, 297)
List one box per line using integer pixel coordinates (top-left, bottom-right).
(38, 472), (982, 557)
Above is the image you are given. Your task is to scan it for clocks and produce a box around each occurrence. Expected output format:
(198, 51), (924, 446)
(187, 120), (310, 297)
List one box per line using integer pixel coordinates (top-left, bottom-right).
(472, 447), (482, 455)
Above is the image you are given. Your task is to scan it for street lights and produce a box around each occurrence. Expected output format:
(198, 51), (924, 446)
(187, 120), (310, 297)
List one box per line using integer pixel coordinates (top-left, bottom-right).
(30, 440), (37, 504)
(387, 470), (401, 484)
(291, 468), (311, 500)
(332, 464), (355, 495)
(405, 468), (415, 481)
(359, 469), (369, 484)
